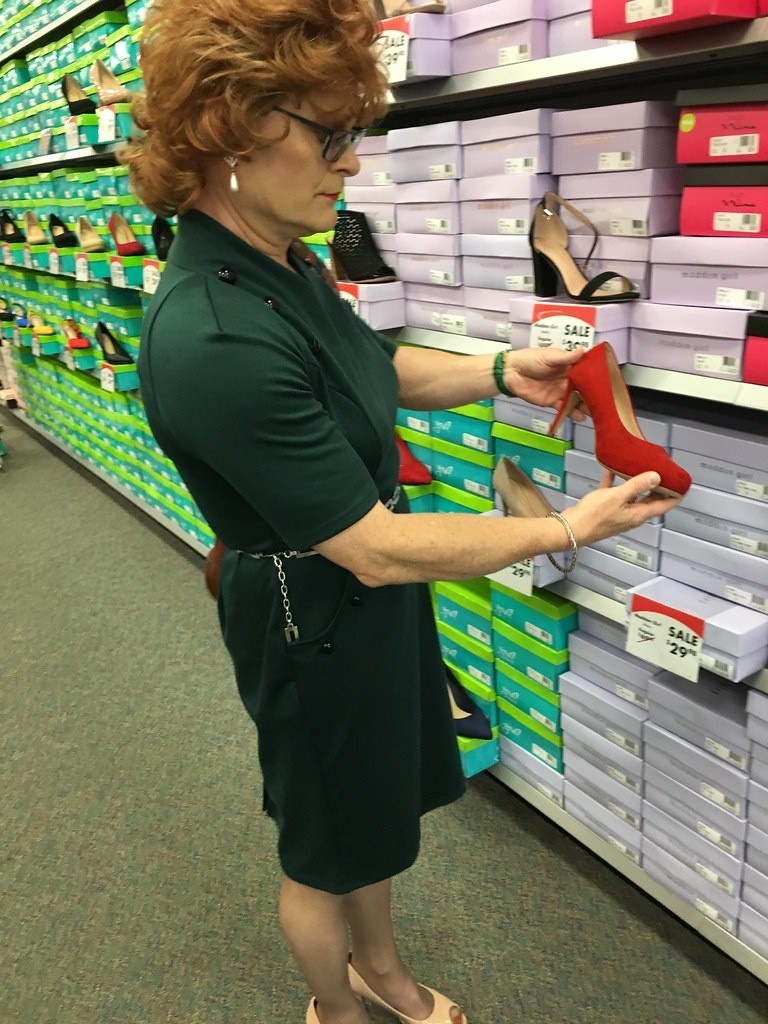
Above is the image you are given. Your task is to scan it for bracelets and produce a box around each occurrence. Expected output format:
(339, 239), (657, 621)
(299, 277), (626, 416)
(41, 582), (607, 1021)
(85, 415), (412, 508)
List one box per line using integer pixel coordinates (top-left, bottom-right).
(493, 349), (516, 398)
(546, 512), (578, 574)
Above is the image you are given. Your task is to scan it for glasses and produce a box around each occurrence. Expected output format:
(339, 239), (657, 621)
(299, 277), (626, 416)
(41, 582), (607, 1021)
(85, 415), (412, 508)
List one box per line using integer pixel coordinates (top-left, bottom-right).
(272, 106), (368, 163)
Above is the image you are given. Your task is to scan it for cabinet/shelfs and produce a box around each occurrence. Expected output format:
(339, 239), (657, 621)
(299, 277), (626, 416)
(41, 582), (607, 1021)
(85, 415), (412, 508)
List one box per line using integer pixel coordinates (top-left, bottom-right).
(0, 0), (768, 986)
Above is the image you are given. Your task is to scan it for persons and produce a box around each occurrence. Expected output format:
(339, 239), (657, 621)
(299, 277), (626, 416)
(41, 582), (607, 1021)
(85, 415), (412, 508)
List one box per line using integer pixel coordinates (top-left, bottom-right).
(119, 1), (683, 1024)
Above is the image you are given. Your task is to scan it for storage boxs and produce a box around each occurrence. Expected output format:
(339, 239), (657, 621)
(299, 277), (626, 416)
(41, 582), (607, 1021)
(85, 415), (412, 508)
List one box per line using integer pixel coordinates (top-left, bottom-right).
(0, 0), (768, 959)
(0, 0), (768, 1024)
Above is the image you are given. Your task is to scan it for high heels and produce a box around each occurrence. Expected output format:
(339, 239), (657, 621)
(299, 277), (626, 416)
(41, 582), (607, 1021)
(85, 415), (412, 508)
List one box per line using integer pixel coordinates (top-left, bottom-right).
(306, 996), (320, 1024)
(493, 455), (555, 519)
(549, 341), (692, 499)
(529, 191), (641, 304)
(89, 59), (130, 106)
(327, 210), (397, 282)
(394, 432), (433, 485)
(347, 951), (468, 1024)
(0, 208), (174, 364)
(62, 74), (96, 115)
(444, 661), (493, 740)
